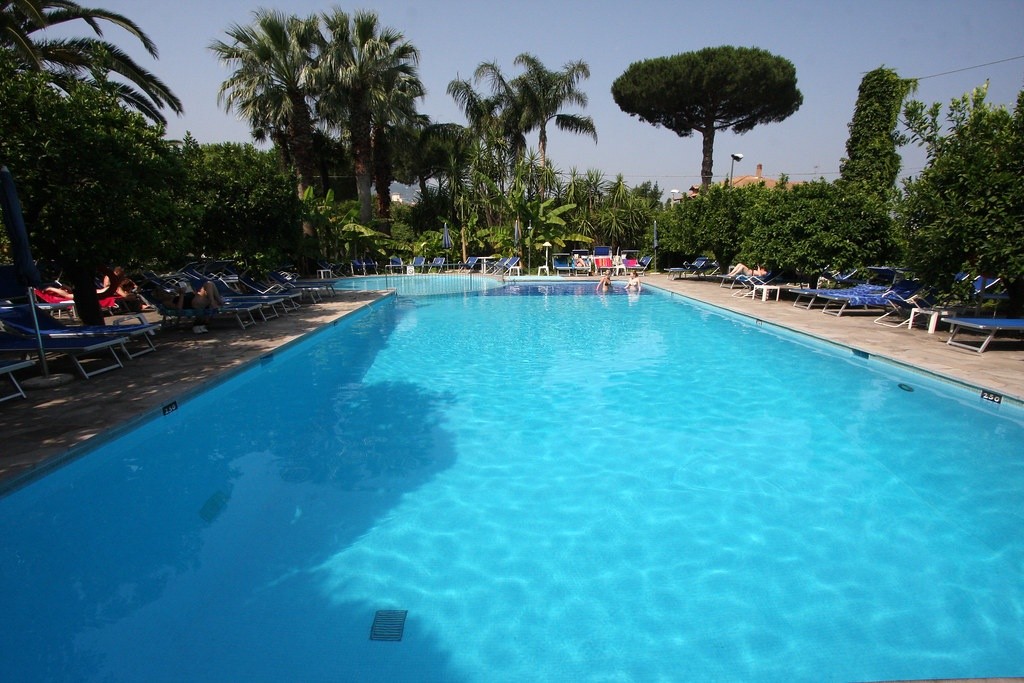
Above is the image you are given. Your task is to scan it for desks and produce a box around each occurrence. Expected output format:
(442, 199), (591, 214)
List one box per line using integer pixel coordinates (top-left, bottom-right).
(112, 314), (155, 337)
(508, 265), (520, 276)
(37, 303), (74, 320)
(316, 270), (331, 279)
(908, 308), (957, 334)
(537, 266), (550, 276)
(752, 285), (800, 302)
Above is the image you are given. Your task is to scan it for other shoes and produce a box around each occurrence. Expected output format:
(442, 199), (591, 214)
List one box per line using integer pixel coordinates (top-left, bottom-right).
(193, 326), (208, 333)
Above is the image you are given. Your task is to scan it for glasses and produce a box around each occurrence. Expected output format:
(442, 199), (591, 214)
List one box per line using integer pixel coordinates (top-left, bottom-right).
(123, 281), (133, 289)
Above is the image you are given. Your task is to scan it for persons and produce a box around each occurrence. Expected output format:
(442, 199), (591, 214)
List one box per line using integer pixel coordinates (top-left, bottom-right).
(158, 281), (226, 312)
(624, 270), (641, 291)
(729, 263), (768, 277)
(596, 270), (614, 294)
(576, 253), (587, 268)
(103, 266), (153, 310)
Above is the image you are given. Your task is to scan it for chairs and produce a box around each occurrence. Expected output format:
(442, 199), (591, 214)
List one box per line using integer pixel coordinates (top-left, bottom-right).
(551, 246), (654, 277)
(385, 256), (446, 274)
(663, 258), (721, 281)
(318, 257), (378, 277)
(714, 265), (1024, 353)
(458, 256), (520, 275)
(0, 260), (338, 407)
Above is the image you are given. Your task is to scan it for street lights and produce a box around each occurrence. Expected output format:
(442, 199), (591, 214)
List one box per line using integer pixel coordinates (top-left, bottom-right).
(730, 152), (744, 193)
